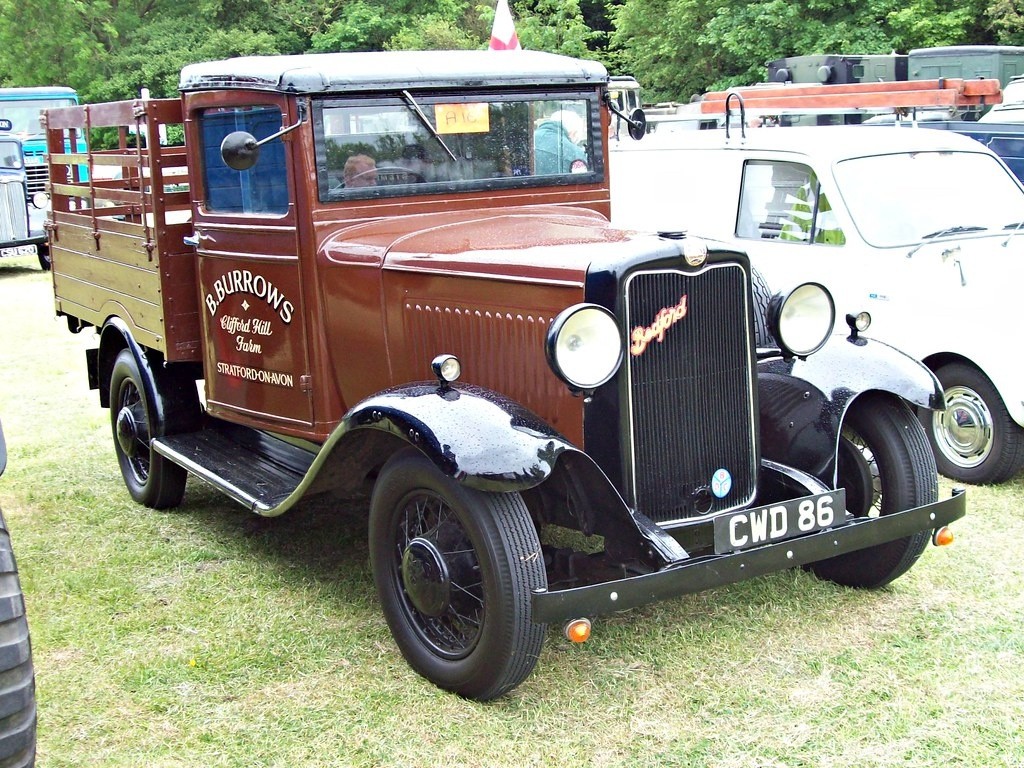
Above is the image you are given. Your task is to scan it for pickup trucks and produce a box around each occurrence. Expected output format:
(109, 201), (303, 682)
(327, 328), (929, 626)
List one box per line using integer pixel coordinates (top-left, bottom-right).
(39, 50), (966, 703)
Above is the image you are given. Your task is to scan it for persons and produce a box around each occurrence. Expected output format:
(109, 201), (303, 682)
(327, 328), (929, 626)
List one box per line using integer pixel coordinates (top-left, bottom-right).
(334, 155), (378, 189)
(533, 111), (588, 173)
(404, 144), (445, 183)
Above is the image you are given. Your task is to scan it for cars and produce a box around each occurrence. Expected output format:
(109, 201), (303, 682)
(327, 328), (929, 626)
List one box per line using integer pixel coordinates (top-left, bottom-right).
(0, 133), (51, 270)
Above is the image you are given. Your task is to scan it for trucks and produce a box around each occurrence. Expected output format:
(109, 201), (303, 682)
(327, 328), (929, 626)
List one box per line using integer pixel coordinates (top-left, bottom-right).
(607, 45), (1024, 186)
(0, 87), (89, 203)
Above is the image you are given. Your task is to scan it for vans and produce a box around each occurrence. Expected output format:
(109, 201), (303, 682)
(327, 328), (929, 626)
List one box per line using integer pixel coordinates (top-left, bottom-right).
(608, 93), (1024, 485)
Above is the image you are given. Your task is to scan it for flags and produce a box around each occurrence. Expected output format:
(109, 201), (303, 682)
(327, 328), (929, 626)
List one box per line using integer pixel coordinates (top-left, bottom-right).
(489, 0), (521, 50)
